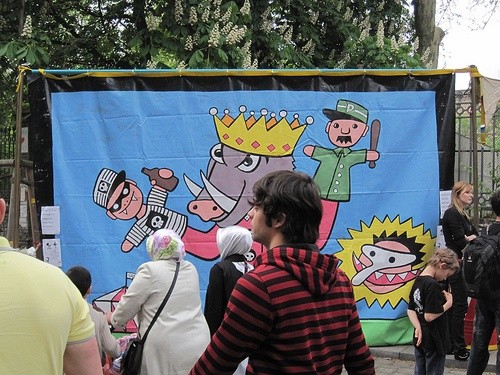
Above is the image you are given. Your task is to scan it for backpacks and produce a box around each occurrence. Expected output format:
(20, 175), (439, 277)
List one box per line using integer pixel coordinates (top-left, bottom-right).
(464, 224), (500, 300)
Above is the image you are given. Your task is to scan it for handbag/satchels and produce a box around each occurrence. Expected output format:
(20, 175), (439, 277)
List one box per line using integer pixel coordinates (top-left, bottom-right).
(120, 338), (144, 372)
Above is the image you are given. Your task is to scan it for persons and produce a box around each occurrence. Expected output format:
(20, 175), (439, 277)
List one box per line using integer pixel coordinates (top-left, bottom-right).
(466, 190), (500, 375)
(105, 229), (211, 375)
(407, 247), (460, 375)
(443, 181), (480, 360)
(188, 171), (377, 375)
(0, 198), (103, 375)
(65, 266), (121, 362)
(202, 225), (256, 375)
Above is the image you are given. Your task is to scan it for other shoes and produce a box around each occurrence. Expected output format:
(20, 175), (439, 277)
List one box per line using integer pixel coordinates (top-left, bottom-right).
(455, 348), (469, 360)
